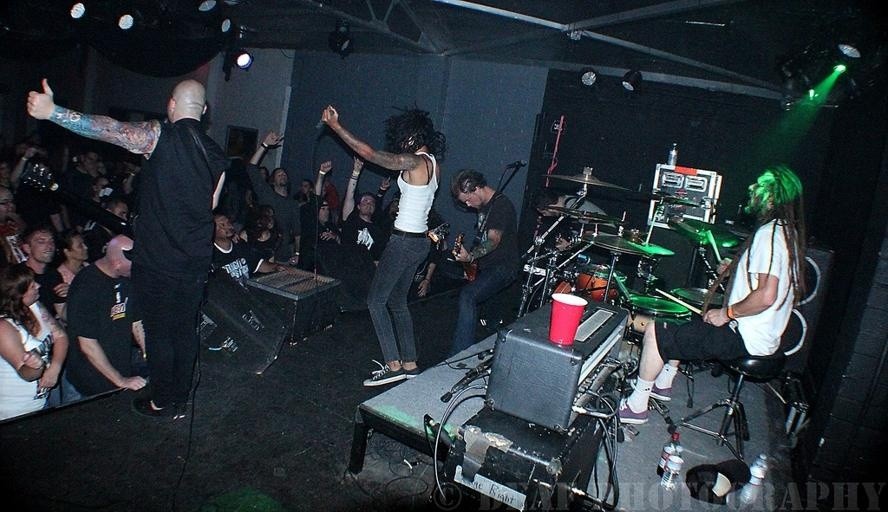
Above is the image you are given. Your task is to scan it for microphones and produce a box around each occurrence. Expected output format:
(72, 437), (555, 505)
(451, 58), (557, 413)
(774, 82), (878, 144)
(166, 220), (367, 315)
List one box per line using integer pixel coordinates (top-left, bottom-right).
(315, 111), (334, 128)
(508, 160), (527, 167)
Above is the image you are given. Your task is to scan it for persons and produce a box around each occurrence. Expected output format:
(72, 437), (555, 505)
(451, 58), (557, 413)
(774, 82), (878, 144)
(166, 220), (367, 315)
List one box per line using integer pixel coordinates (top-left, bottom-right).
(323, 101), (446, 386)
(616, 163), (806, 425)
(1, 132), (447, 420)
(26, 78), (231, 415)
(448, 167), (522, 359)
(527, 185), (618, 266)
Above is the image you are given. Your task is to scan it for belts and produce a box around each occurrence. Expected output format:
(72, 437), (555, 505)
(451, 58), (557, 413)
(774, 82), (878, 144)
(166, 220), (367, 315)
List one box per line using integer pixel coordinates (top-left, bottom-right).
(391, 226), (432, 240)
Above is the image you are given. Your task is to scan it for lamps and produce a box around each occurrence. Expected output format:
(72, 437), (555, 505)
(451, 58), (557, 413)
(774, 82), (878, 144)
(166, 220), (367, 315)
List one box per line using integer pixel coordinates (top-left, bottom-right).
(837, 35), (869, 61)
(62, 0), (85, 22)
(578, 67), (597, 88)
(214, 20), (235, 35)
(326, 29), (352, 55)
(232, 49), (253, 72)
(192, 0), (220, 16)
(111, 8), (135, 32)
(620, 67), (642, 91)
(830, 59), (849, 75)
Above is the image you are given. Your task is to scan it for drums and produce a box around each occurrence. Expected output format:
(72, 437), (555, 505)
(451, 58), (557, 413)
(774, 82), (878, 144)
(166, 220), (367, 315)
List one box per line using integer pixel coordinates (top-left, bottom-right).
(551, 281), (573, 295)
(626, 293), (693, 337)
(577, 264), (624, 302)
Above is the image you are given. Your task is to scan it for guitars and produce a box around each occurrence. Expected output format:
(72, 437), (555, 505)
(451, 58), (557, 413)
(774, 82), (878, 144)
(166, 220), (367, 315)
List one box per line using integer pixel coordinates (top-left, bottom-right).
(453, 234), (481, 283)
(20, 164), (135, 259)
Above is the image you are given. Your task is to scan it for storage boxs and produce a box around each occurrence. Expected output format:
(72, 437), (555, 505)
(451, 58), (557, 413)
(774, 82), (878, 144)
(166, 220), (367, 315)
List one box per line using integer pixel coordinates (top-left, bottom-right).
(647, 164), (723, 231)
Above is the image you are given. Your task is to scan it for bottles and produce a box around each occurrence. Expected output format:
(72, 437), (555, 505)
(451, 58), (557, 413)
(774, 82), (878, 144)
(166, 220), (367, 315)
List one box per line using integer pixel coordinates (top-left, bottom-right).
(667, 144), (677, 165)
(661, 446), (684, 492)
(738, 454), (768, 504)
(657, 432), (681, 478)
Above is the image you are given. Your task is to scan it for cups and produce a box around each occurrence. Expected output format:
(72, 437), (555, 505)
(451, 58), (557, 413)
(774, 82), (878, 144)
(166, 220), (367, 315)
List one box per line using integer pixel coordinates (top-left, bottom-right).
(549, 293), (589, 349)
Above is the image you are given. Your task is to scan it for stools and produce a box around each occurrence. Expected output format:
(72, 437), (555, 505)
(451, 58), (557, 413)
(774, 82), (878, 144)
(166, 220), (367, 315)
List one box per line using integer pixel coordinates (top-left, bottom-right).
(667, 356), (781, 460)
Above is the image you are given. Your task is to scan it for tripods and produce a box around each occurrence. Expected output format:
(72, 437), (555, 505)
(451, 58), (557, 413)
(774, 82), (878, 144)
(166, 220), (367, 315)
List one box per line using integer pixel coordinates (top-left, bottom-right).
(440, 197), (593, 404)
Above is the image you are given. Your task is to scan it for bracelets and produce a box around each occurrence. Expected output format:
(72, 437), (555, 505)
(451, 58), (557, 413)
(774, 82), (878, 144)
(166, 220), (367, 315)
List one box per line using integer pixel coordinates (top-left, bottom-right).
(727, 306), (736, 320)
(720, 307), (728, 323)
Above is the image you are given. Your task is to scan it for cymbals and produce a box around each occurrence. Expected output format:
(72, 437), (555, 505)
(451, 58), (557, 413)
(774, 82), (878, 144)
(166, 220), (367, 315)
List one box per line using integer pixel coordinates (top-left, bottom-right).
(635, 240), (674, 257)
(542, 167), (627, 190)
(671, 216), (738, 251)
(542, 204), (620, 225)
(671, 286), (724, 307)
(582, 236), (640, 257)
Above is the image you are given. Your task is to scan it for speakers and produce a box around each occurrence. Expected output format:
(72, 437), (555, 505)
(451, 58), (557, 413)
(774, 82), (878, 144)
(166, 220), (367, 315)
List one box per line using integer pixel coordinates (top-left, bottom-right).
(776, 244), (840, 377)
(302, 235), (377, 312)
(197, 267), (288, 375)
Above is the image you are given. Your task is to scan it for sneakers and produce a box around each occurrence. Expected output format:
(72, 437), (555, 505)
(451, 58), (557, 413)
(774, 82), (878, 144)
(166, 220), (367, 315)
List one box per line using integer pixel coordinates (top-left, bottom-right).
(615, 398), (650, 426)
(130, 397), (178, 422)
(363, 367), (407, 387)
(629, 378), (673, 402)
(400, 360), (423, 379)
